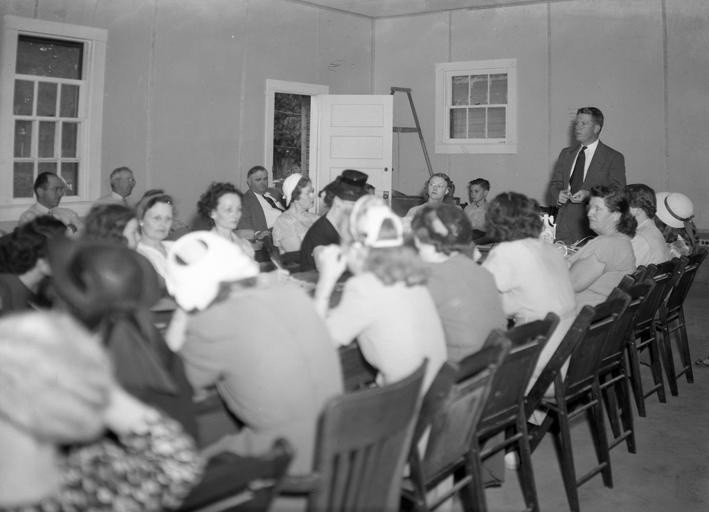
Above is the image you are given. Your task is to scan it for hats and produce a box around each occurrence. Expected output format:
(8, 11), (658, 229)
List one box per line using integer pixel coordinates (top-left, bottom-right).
(282, 173), (303, 207)
(51, 241), (162, 317)
(328, 170), (374, 200)
(421, 204), (472, 245)
(655, 191), (694, 229)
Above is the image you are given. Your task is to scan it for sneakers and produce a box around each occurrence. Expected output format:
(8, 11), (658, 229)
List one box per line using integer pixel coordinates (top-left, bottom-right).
(505, 452), (521, 471)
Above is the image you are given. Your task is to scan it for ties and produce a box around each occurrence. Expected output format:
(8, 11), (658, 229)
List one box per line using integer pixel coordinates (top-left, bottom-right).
(569, 146), (588, 195)
(263, 195), (284, 213)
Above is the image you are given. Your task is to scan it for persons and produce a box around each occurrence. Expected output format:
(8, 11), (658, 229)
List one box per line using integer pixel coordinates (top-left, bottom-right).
(1, 164), (694, 512)
(544, 105), (626, 243)
